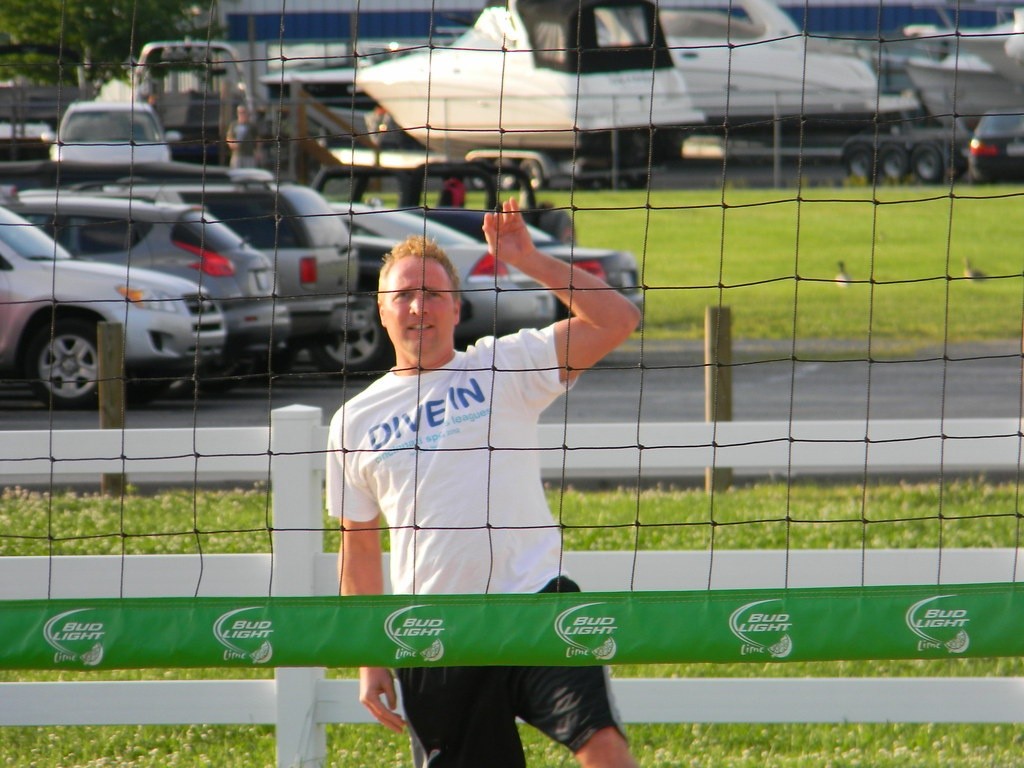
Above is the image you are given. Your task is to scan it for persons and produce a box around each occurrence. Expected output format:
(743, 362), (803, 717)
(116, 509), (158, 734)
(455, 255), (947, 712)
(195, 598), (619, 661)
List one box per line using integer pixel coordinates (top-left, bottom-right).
(324, 198), (642, 768)
(225, 105), (292, 174)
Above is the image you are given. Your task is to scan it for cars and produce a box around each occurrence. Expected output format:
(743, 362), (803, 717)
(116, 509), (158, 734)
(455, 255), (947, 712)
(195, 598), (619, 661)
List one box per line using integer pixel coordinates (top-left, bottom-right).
(0, 0), (1024, 408)
